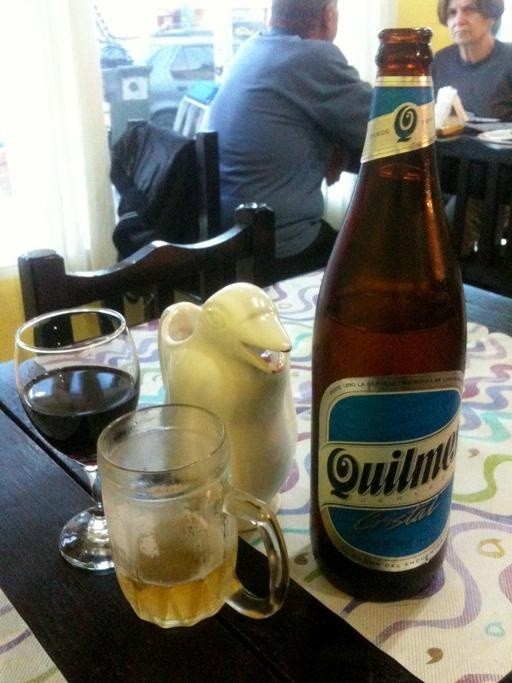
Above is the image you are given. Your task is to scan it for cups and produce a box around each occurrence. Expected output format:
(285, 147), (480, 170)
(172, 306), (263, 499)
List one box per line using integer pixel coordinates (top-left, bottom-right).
(99, 404), (288, 630)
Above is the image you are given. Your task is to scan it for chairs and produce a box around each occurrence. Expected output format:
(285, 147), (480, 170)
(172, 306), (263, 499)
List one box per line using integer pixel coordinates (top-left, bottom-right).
(111, 120), (222, 260)
(16, 198), (279, 355)
(434, 134), (511, 297)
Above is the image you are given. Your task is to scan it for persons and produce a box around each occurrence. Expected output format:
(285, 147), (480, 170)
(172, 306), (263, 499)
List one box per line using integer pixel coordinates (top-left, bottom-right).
(431, 1), (512, 259)
(199, 0), (373, 281)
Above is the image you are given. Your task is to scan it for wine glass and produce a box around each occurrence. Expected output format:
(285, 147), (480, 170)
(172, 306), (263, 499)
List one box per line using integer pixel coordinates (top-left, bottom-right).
(13, 306), (140, 573)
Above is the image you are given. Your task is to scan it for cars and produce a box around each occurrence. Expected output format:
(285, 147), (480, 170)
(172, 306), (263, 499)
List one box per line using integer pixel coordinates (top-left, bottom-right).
(98, 17), (270, 152)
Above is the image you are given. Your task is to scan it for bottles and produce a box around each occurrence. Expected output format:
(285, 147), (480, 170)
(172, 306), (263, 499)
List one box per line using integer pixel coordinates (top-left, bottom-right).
(309, 27), (466, 604)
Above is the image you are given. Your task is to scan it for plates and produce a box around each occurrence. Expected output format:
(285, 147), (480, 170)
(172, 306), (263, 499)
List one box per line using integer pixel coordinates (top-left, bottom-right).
(478, 128), (512, 151)
